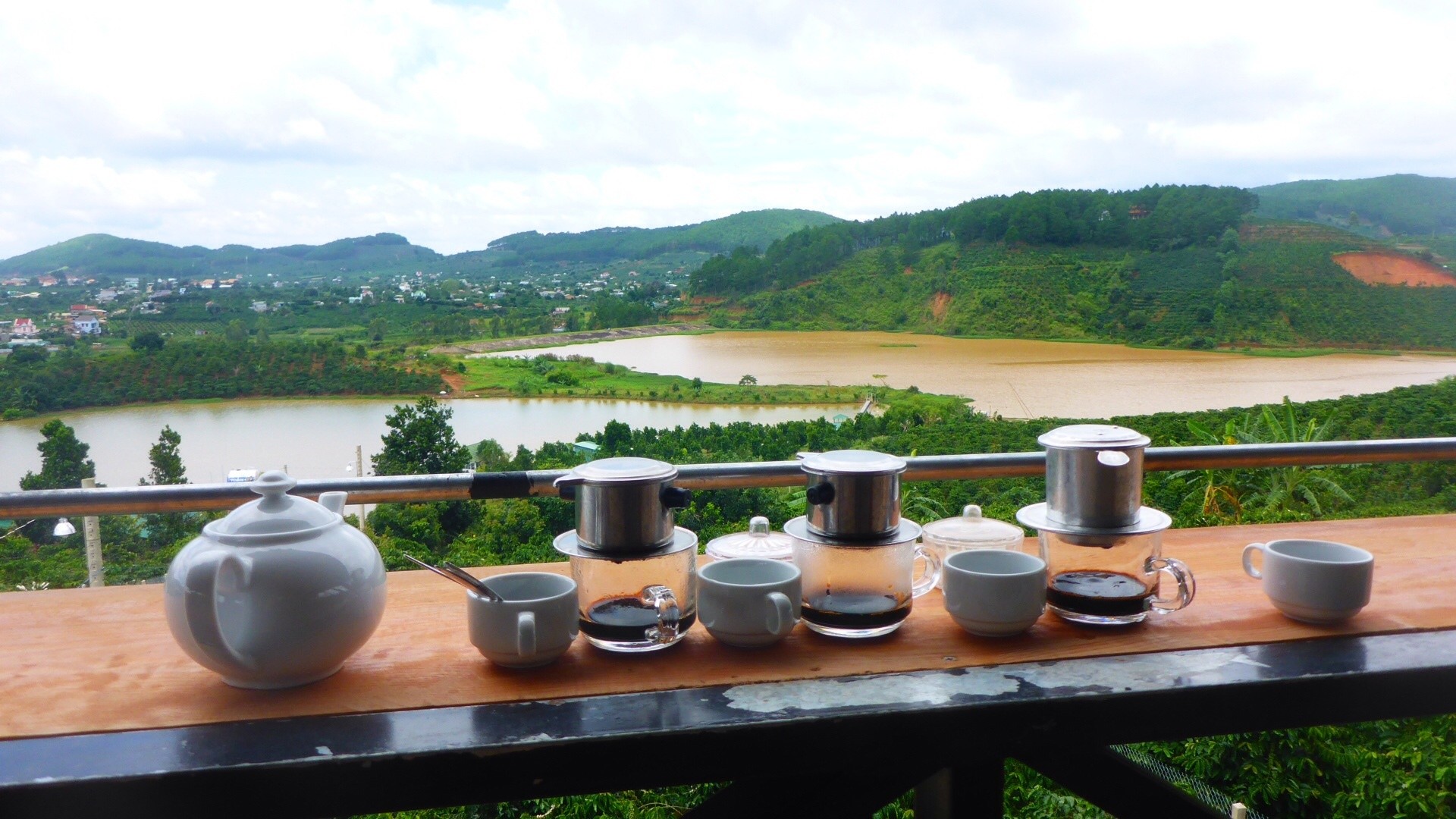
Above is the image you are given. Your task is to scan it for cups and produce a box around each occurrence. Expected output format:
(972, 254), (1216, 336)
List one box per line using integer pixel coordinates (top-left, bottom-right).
(701, 561), (801, 647)
(1039, 423), (1150, 527)
(552, 526), (700, 653)
(468, 572), (579, 665)
(1015, 502), (1194, 626)
(941, 551), (1048, 636)
(795, 447), (906, 543)
(1244, 540), (1374, 625)
(782, 514), (936, 640)
(554, 456), (693, 560)
(924, 502), (1024, 596)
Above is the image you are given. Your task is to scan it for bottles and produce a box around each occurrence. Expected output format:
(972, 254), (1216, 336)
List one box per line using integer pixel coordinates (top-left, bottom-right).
(705, 517), (793, 561)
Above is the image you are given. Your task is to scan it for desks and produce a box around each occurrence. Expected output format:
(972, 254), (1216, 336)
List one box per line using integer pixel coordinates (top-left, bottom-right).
(0, 514), (1455, 818)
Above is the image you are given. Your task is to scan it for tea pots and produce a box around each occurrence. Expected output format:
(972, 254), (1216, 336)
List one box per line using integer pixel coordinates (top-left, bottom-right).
(162, 471), (385, 688)
